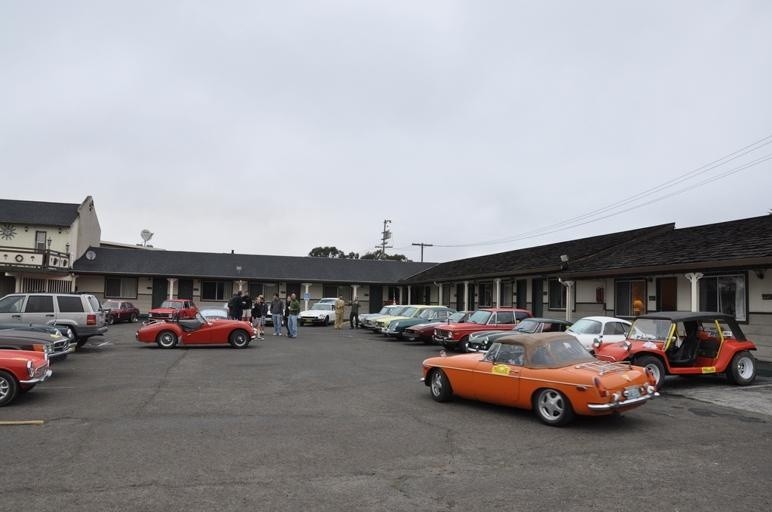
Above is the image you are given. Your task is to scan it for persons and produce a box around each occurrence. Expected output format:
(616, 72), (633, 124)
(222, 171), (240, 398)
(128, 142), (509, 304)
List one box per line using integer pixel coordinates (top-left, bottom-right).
(346, 296), (363, 329)
(223, 288), (301, 340)
(632, 287), (647, 318)
(332, 294), (345, 330)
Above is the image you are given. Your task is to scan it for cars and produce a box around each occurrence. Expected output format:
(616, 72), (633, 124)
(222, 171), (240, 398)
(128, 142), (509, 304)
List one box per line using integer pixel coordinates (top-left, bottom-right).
(1, 298), (141, 405)
(588, 309), (758, 393)
(145, 298), (197, 320)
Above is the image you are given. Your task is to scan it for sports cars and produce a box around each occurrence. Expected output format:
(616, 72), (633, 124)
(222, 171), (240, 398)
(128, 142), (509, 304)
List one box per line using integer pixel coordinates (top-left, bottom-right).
(133, 309), (259, 352)
(414, 329), (660, 429)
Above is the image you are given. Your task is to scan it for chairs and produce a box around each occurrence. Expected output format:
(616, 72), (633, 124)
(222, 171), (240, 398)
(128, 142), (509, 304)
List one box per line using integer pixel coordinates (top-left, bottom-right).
(668, 336), (719, 367)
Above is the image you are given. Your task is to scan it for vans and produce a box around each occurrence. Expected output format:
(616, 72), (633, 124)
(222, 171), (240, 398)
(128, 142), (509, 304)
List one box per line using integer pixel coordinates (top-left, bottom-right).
(1, 292), (109, 347)
